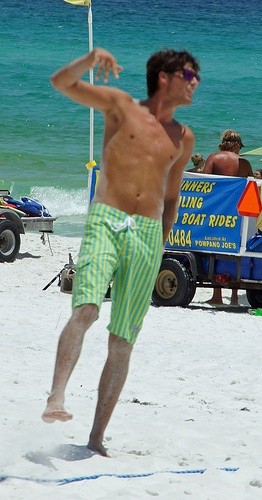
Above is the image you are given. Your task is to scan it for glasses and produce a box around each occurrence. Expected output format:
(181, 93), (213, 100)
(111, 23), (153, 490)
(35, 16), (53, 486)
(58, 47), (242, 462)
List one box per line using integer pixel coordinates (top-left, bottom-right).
(172, 68), (201, 84)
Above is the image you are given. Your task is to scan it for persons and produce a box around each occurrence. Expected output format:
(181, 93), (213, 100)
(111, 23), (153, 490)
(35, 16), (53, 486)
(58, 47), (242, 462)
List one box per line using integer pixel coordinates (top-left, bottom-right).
(203, 131), (253, 305)
(254, 169), (262, 180)
(187, 153), (205, 173)
(42, 46), (201, 458)
